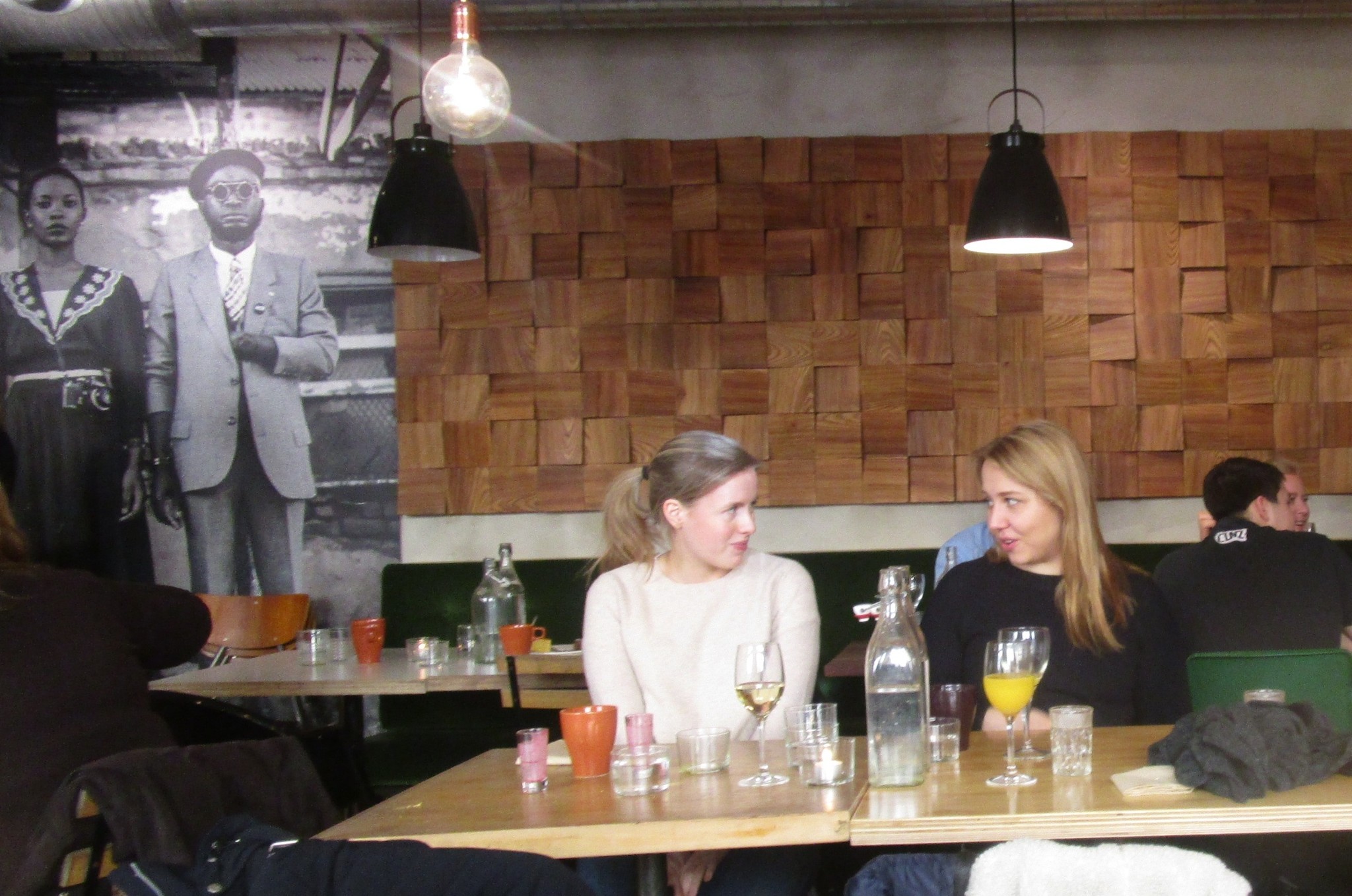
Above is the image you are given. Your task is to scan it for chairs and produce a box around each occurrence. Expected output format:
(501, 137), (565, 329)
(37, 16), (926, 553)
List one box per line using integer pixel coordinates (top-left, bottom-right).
(1185, 647), (1352, 738)
(490, 655), (594, 733)
(194, 593), (314, 724)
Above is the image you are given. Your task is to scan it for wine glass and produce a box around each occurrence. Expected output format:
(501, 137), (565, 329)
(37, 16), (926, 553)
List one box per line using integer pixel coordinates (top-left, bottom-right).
(983, 625), (1051, 787)
(734, 642), (790, 787)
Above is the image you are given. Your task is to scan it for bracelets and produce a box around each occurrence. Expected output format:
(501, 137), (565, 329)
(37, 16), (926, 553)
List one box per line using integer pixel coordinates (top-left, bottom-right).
(125, 440), (145, 451)
(152, 457), (171, 464)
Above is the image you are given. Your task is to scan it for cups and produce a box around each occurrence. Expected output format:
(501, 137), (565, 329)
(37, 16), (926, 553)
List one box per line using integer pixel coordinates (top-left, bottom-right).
(610, 744), (671, 796)
(560, 705), (618, 778)
(296, 629), (331, 666)
(351, 618), (386, 663)
(785, 701), (857, 786)
(910, 574), (926, 612)
(417, 641), (450, 665)
(405, 636), (439, 662)
(516, 728), (549, 794)
(326, 627), (349, 662)
(500, 625), (546, 655)
(531, 639), (552, 653)
(457, 624), (474, 658)
(930, 683), (979, 752)
(928, 719), (962, 763)
(1245, 688), (1286, 704)
(1048, 705), (1094, 777)
(625, 712), (654, 781)
(677, 727), (731, 776)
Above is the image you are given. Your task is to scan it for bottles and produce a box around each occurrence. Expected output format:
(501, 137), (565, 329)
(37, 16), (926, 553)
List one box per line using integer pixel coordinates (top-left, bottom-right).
(496, 543), (527, 665)
(1303, 523), (1314, 533)
(935, 547), (958, 586)
(471, 558), (518, 665)
(865, 566), (930, 788)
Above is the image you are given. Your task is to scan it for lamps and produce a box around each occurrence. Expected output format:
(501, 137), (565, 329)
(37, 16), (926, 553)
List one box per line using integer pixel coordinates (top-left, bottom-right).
(366, 0), (482, 263)
(963, 0), (1073, 254)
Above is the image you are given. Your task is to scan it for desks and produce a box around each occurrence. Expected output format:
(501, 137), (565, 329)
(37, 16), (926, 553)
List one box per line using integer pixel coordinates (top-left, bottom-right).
(309, 730), (863, 896)
(850, 725), (1352, 896)
(145, 643), (586, 810)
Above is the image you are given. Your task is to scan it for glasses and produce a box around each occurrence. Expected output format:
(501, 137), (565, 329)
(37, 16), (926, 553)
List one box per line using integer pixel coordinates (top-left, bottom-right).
(199, 181), (258, 201)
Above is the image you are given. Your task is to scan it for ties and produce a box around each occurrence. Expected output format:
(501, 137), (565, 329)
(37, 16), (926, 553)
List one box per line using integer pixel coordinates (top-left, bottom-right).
(223, 259), (247, 334)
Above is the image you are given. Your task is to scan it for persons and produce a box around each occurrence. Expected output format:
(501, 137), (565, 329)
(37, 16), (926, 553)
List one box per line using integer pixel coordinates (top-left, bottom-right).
(145, 152), (340, 596)
(575, 431), (823, 896)
(916, 422), (1194, 725)
(1151, 457), (1352, 729)
(0, 163), (154, 592)
